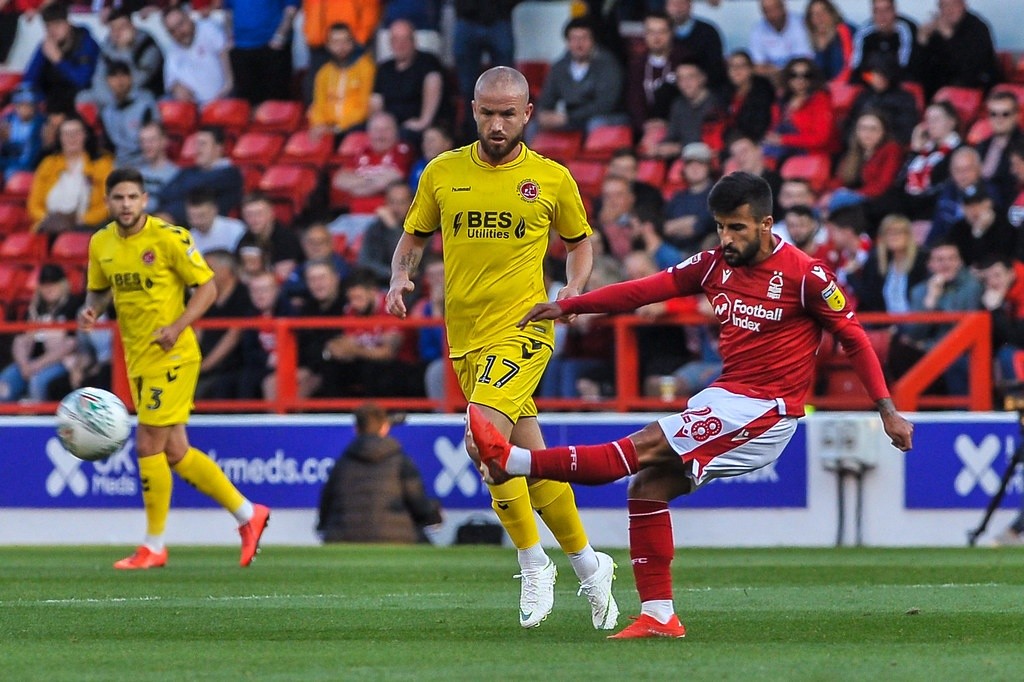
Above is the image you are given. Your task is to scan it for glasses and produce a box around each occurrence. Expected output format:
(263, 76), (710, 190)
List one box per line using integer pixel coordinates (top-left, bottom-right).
(787, 70), (812, 81)
(990, 110), (1012, 118)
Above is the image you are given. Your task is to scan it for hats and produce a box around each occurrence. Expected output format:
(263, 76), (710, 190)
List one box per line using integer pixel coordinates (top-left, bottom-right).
(38, 264), (63, 283)
(681, 143), (711, 164)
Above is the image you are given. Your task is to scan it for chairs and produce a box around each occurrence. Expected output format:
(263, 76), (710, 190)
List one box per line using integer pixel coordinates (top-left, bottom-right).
(0, 53), (1024, 396)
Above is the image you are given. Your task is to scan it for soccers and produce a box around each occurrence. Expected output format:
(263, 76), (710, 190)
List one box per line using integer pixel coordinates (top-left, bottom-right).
(56, 387), (132, 462)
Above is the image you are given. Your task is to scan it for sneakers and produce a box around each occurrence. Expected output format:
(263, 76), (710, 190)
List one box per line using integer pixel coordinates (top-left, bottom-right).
(576, 552), (621, 629)
(464, 404), (515, 485)
(606, 613), (686, 638)
(114, 546), (167, 570)
(236, 504), (271, 567)
(513, 554), (557, 629)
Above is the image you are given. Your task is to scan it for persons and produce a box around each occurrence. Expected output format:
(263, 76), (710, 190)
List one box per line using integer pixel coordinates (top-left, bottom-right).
(316, 401), (442, 546)
(0, 0), (518, 419)
(75, 167), (271, 568)
(467, 170), (915, 641)
(529, 0), (1024, 413)
(374, 18), (448, 141)
(533, 17), (624, 130)
(305, 23), (378, 136)
(91, 61), (164, 160)
(383, 64), (620, 631)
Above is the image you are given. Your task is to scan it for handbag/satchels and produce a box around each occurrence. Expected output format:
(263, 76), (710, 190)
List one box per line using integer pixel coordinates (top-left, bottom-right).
(456, 518), (504, 546)
